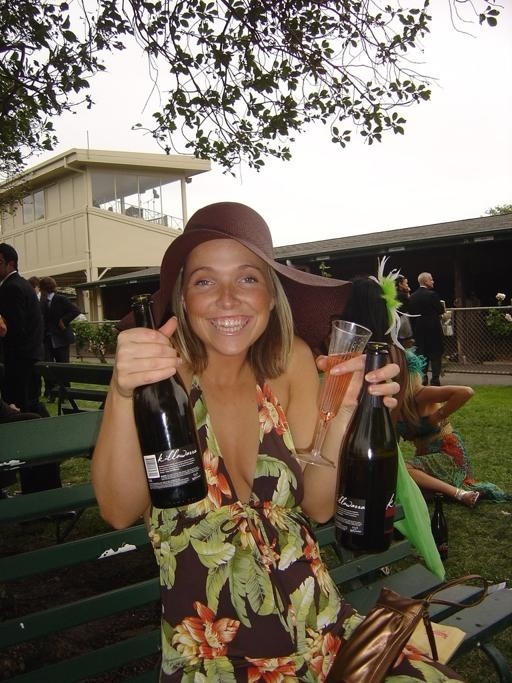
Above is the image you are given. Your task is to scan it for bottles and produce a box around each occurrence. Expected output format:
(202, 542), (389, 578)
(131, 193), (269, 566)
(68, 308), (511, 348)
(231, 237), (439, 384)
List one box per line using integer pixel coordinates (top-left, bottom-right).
(333, 343), (399, 557)
(130, 293), (208, 510)
(432, 492), (449, 566)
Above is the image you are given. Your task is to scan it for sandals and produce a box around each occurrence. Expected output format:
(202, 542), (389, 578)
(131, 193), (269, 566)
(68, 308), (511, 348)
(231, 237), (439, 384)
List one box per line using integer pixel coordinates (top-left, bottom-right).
(455, 488), (479, 508)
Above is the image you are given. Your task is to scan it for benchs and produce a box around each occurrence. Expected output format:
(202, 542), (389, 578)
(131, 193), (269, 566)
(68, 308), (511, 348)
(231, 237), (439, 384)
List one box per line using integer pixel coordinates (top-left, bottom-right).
(0, 362), (511, 682)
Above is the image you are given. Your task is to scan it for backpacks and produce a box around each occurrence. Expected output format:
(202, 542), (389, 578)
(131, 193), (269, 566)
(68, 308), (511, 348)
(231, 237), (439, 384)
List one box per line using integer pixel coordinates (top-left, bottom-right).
(399, 315), (413, 338)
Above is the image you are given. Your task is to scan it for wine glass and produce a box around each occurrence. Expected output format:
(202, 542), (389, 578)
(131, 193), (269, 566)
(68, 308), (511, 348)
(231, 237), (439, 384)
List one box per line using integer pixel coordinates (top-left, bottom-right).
(289, 315), (372, 475)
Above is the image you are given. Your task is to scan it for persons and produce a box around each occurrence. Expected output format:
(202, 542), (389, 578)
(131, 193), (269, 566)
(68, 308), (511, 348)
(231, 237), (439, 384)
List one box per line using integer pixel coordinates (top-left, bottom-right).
(0, 242), (90, 524)
(90, 199), (466, 683)
(342, 268), (481, 577)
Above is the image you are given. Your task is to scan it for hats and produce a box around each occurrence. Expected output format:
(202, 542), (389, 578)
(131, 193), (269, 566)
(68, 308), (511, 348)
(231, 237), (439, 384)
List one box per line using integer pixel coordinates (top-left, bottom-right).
(114, 202), (353, 373)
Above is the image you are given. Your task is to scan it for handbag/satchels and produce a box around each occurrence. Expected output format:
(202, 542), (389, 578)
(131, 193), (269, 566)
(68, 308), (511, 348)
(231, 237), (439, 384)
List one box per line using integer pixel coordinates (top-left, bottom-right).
(439, 309), (454, 336)
(324, 588), (428, 683)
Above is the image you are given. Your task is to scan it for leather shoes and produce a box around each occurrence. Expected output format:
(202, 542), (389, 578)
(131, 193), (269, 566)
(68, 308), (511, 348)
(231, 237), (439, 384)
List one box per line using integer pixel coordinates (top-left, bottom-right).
(19, 511), (76, 525)
